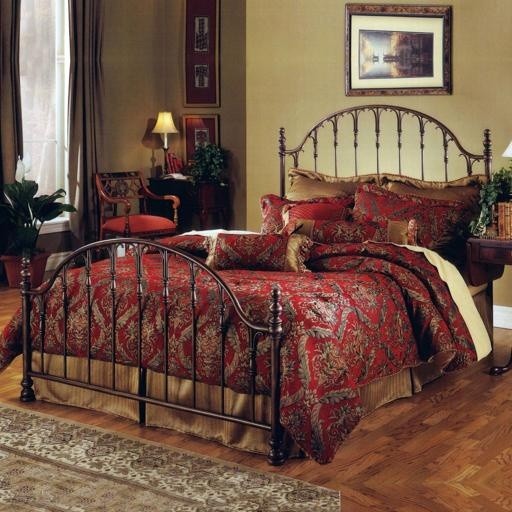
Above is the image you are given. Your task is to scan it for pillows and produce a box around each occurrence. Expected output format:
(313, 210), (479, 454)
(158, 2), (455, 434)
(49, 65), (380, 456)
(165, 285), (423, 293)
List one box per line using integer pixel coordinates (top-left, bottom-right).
(260, 169), (486, 245)
(206, 232), (312, 276)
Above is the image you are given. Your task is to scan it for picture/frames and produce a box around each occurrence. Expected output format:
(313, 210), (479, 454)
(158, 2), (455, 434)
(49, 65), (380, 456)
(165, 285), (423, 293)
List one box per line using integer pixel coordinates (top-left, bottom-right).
(343, 2), (454, 97)
(183, 115), (220, 168)
(184, 0), (220, 108)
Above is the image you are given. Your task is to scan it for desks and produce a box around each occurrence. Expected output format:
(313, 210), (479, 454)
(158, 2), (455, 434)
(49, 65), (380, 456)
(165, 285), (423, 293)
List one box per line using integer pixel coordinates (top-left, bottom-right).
(150, 178), (231, 228)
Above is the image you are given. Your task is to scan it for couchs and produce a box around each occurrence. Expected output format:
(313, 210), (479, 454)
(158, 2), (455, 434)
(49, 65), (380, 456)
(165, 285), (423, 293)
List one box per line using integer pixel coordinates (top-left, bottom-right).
(96, 170), (182, 257)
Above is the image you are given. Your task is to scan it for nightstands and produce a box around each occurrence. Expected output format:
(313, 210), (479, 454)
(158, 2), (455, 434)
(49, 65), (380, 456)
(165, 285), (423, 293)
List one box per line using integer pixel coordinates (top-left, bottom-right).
(467, 236), (511, 375)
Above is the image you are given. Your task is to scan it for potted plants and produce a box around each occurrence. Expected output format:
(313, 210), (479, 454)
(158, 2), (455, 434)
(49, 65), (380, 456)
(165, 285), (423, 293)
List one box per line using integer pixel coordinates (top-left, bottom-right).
(470, 166), (512, 239)
(0, 178), (79, 289)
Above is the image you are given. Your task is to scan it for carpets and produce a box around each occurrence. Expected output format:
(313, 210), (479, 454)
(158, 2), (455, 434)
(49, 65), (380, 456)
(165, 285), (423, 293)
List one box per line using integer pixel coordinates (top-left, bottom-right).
(0, 405), (343, 512)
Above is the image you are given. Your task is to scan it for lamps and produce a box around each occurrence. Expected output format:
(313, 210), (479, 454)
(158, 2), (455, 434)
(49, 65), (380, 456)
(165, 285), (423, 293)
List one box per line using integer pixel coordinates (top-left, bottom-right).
(501, 138), (512, 160)
(151, 111), (180, 174)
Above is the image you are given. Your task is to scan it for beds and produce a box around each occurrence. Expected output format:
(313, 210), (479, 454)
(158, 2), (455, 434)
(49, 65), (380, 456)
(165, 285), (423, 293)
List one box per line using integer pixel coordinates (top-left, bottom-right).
(19, 105), (491, 466)
